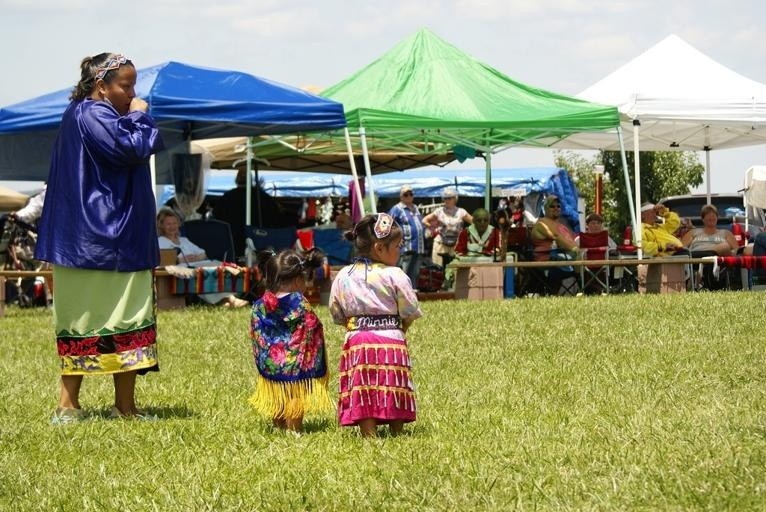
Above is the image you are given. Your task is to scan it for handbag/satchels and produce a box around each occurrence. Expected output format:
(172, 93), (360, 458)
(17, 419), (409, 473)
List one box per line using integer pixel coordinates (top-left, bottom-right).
(416, 260), (444, 292)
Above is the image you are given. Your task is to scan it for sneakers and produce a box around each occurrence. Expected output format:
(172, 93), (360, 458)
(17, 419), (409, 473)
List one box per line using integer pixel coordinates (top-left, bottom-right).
(52, 406), (85, 425)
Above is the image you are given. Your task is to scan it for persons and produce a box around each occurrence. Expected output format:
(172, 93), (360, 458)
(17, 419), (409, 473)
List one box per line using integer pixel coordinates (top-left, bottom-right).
(33, 53), (167, 425)
(389, 184), (739, 296)
(330, 213), (423, 438)
(156, 206), (250, 308)
(250, 248), (329, 433)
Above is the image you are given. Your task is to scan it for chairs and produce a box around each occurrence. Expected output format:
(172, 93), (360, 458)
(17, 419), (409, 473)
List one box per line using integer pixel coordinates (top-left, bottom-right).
(503, 247), (581, 296)
(606, 244), (731, 291)
(578, 229), (610, 294)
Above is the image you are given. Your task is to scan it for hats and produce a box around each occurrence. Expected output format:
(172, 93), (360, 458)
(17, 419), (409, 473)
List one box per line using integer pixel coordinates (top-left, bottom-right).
(400, 187), (412, 198)
(544, 194), (560, 206)
(442, 189), (458, 201)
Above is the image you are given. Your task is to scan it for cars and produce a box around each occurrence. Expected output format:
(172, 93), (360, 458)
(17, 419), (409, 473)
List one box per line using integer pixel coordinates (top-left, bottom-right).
(624, 193), (766, 290)
